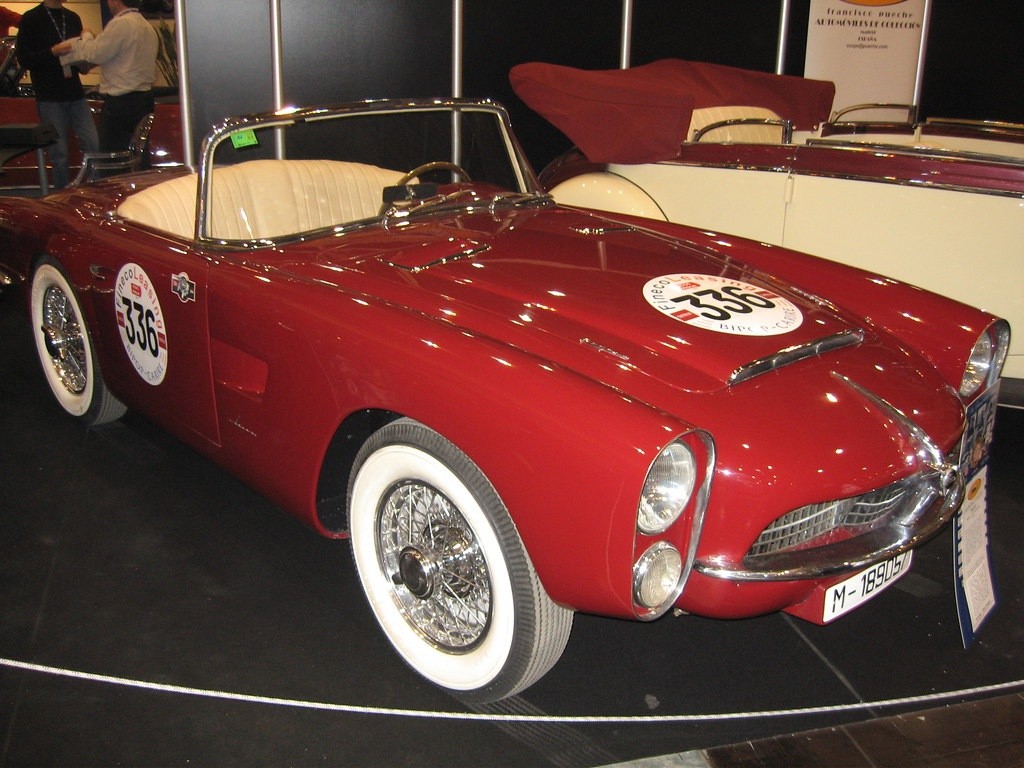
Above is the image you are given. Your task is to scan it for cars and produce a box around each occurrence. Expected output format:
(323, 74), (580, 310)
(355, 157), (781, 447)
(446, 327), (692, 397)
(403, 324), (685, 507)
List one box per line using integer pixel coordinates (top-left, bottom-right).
(508, 60), (1024, 409)
(2, 98), (1012, 710)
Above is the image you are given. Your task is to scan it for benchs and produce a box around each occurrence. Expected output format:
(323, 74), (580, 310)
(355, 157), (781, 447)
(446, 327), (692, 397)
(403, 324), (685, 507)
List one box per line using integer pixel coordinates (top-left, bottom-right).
(118, 158), (426, 245)
(685, 104), (797, 147)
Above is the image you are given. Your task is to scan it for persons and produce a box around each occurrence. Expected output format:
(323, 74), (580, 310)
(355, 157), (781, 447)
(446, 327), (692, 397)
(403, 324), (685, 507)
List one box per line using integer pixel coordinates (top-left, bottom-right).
(16, 0), (103, 192)
(80, 0), (160, 177)
